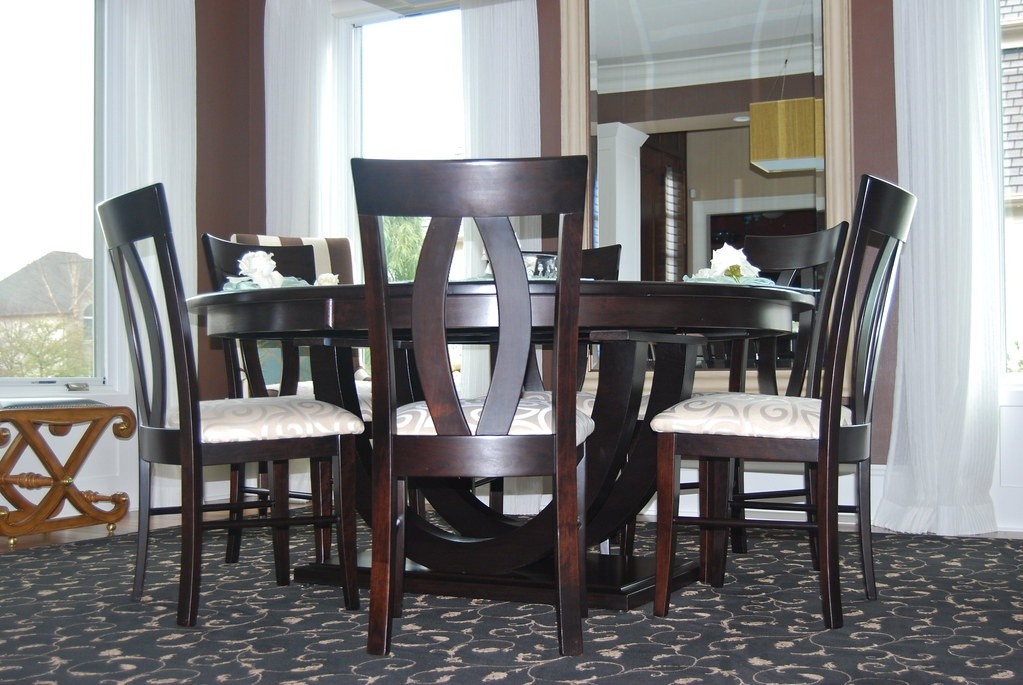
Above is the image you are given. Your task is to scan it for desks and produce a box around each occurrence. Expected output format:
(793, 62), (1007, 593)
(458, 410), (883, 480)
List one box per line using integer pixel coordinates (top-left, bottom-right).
(187, 280), (815, 616)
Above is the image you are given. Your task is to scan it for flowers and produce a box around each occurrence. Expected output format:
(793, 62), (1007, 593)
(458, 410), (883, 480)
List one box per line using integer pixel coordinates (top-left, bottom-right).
(237, 249), (282, 288)
(710, 243), (754, 284)
(311, 273), (339, 286)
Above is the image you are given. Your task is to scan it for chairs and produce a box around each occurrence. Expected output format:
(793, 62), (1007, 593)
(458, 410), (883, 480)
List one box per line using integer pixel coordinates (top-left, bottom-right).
(95, 155), (917, 650)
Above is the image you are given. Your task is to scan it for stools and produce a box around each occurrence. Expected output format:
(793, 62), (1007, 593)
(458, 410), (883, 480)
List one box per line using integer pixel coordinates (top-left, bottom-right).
(0, 398), (136, 550)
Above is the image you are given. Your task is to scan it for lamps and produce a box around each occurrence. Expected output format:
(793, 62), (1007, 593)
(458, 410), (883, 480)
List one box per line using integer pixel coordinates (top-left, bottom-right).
(749, 97), (824, 174)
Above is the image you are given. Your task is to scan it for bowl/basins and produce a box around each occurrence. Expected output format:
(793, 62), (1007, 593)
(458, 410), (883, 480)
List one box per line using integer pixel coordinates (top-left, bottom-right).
(521, 253), (558, 280)
